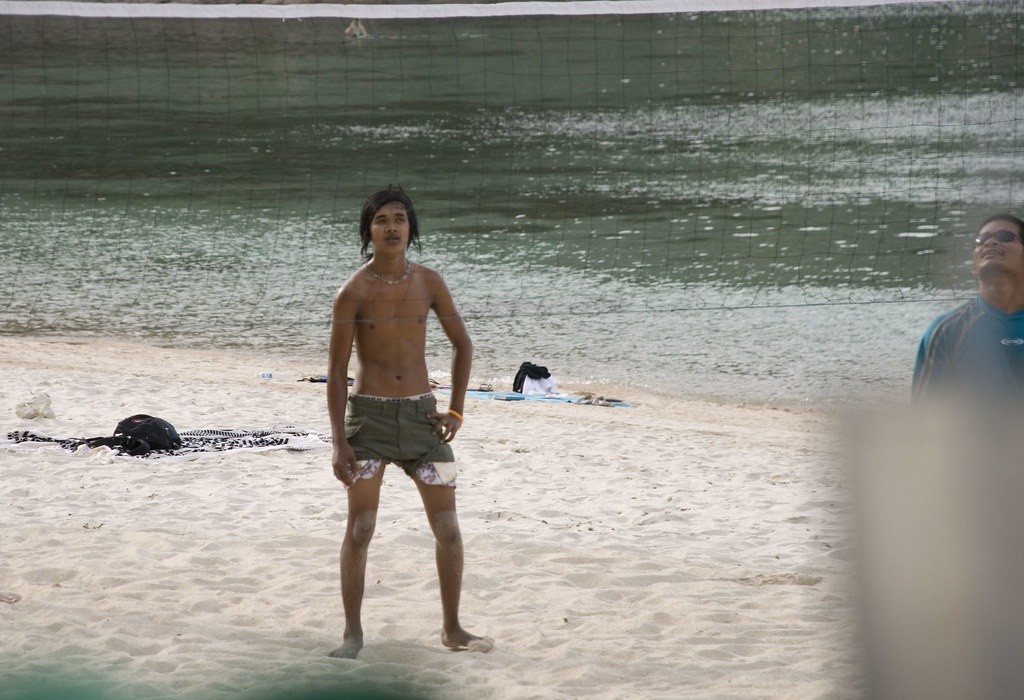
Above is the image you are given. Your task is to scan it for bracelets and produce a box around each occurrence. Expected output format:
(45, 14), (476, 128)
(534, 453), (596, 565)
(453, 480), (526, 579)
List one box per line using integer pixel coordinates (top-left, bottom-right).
(447, 410), (464, 421)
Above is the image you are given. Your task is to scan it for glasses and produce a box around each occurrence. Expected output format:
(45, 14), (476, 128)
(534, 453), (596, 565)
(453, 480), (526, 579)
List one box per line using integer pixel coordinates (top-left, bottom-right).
(975, 230), (1024, 246)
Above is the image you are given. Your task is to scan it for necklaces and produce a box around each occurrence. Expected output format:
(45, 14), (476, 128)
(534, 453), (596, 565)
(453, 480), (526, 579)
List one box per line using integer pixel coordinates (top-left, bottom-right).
(362, 257), (411, 285)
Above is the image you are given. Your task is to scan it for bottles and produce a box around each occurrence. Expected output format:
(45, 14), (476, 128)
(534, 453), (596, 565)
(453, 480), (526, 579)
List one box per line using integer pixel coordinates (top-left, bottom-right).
(260, 372), (277, 378)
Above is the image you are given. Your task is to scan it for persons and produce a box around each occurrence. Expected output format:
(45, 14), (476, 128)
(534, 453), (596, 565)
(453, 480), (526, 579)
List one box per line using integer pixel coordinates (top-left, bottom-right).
(911, 214), (1024, 411)
(327, 184), (493, 659)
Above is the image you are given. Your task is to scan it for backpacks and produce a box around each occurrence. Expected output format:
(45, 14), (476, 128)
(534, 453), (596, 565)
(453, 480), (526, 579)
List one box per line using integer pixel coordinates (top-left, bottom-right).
(85, 414), (181, 455)
